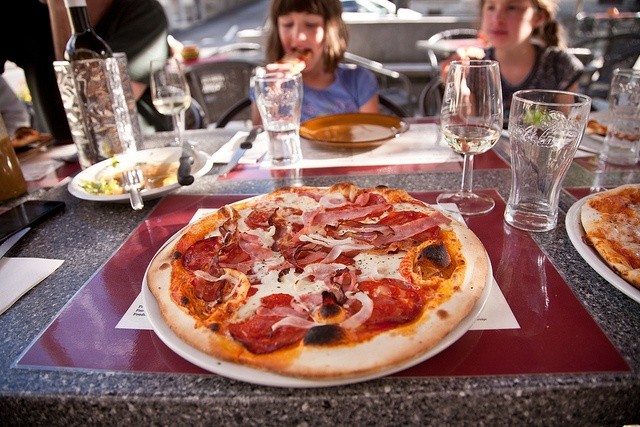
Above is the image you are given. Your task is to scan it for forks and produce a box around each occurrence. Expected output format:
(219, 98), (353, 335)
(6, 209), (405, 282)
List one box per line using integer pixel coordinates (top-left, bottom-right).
(122, 169), (145, 211)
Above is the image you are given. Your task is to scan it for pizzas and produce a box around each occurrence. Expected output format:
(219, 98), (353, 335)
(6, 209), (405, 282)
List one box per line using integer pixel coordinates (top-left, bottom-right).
(579, 183), (640, 290)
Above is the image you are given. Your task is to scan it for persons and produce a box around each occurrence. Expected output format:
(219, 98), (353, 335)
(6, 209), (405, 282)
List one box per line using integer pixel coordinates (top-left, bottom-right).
(251, 0), (380, 128)
(46, 1), (168, 134)
(440, 0), (584, 117)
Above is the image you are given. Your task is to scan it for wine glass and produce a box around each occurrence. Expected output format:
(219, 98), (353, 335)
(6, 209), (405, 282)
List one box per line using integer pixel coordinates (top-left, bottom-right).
(437, 59), (504, 215)
(150, 59), (201, 148)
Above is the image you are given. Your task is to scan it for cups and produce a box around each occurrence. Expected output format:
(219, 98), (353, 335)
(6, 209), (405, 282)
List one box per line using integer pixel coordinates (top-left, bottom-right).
(493, 219), (550, 336)
(0, 115), (27, 201)
(254, 73), (304, 166)
(504, 90), (592, 232)
(600, 68), (636, 165)
(592, 154), (635, 197)
(54, 53), (145, 171)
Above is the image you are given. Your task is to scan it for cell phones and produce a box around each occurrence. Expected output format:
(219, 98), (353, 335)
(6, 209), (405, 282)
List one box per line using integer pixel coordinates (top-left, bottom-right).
(0, 200), (65, 244)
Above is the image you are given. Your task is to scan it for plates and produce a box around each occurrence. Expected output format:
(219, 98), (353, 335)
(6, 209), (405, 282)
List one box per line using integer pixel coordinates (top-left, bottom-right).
(300, 112), (409, 148)
(564, 183), (639, 304)
(68, 146), (212, 200)
(209, 132), (271, 164)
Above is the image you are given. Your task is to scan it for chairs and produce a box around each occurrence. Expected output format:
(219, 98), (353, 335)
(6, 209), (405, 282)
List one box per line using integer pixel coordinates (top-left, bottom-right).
(426, 28), (478, 113)
(215, 95), (406, 128)
(183, 61), (256, 123)
(419, 77), (598, 116)
(572, 34), (640, 98)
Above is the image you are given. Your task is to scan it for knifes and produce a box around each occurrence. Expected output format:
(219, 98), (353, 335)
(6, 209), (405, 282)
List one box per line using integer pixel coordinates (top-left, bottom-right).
(0, 228), (29, 257)
(217, 126), (264, 176)
(178, 134), (195, 185)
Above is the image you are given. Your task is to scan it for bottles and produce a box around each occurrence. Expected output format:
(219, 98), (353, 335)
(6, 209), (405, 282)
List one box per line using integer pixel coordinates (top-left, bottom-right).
(62, 0), (137, 164)
